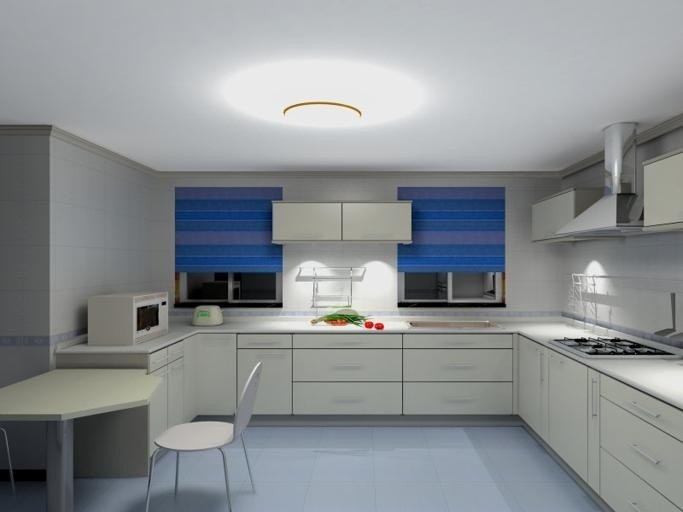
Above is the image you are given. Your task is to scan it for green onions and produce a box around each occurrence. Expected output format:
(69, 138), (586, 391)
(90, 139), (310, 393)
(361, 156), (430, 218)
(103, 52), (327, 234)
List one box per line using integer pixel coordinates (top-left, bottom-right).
(311, 313), (374, 327)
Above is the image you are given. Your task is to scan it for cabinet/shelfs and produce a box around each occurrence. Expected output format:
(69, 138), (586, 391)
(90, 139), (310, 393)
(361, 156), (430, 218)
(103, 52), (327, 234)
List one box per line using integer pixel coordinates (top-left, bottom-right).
(235, 334), (292, 415)
(402, 334), (513, 415)
(518, 334), (600, 501)
(55, 339), (184, 478)
(529, 183), (627, 241)
(271, 199), (414, 246)
(601, 371), (683, 511)
(291, 333), (402, 415)
(642, 147), (683, 234)
(184, 332), (235, 423)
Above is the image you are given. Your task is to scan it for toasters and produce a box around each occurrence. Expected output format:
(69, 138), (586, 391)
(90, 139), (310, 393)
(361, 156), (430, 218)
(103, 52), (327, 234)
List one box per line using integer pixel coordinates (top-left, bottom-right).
(191, 305), (223, 326)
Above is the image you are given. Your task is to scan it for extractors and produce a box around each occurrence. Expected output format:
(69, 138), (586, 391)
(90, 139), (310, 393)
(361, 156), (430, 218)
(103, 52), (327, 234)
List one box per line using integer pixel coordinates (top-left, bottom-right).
(555, 124), (644, 243)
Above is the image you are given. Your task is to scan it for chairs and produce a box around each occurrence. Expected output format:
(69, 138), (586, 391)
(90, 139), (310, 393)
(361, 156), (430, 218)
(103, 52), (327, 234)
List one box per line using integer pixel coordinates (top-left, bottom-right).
(143, 362), (264, 512)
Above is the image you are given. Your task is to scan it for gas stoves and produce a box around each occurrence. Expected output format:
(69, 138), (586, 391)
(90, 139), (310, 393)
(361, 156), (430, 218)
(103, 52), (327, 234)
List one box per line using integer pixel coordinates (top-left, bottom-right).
(554, 337), (677, 358)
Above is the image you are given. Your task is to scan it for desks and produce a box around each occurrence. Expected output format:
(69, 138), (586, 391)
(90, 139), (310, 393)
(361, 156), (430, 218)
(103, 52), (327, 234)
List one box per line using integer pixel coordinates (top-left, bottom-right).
(0, 368), (162, 512)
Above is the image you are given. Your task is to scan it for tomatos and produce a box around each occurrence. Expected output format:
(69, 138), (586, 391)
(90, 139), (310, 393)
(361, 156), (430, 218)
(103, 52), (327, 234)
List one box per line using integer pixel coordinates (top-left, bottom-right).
(375, 323), (383, 329)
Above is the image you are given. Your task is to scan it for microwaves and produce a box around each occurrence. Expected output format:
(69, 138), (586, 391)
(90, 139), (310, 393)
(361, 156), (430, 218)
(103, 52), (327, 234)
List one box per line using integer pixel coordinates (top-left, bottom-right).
(85, 289), (168, 345)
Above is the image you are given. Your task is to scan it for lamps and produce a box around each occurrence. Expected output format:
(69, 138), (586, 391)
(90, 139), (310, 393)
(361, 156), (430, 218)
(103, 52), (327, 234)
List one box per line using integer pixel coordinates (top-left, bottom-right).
(283, 102), (360, 129)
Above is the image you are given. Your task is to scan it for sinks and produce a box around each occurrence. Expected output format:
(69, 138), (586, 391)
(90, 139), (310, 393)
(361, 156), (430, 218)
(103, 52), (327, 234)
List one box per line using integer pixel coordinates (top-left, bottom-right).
(404, 318), (498, 330)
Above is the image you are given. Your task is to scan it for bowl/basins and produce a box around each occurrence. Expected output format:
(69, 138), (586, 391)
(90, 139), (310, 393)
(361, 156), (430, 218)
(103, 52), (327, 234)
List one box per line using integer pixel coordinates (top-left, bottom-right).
(324, 319), (348, 326)
